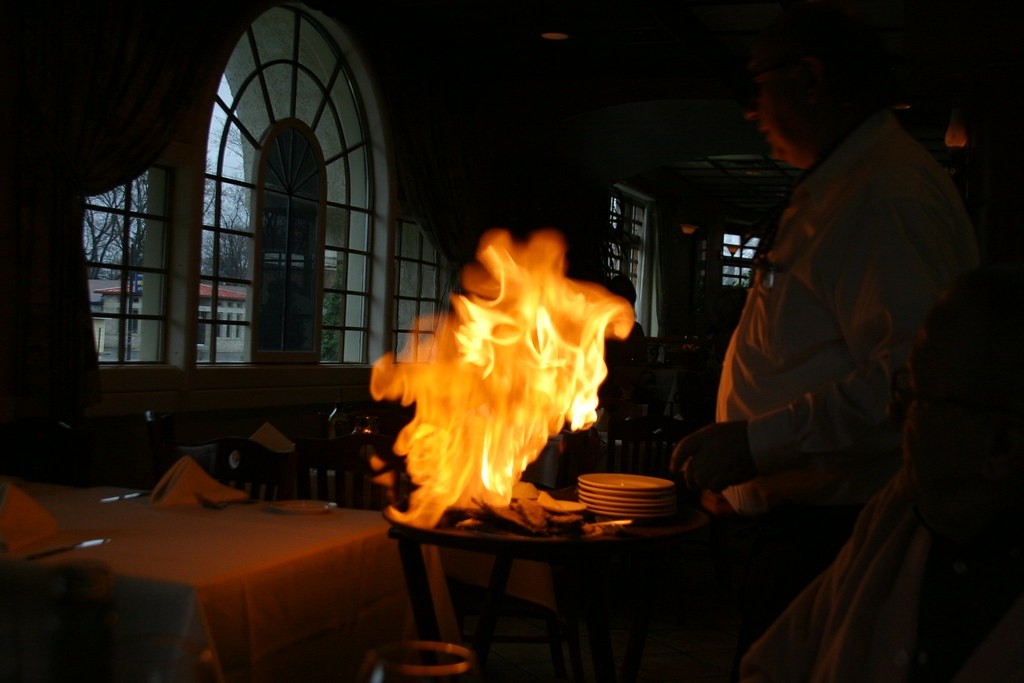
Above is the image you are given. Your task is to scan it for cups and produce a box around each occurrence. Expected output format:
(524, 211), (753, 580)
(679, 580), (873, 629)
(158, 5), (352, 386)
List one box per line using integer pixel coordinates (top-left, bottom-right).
(363, 416), (380, 433)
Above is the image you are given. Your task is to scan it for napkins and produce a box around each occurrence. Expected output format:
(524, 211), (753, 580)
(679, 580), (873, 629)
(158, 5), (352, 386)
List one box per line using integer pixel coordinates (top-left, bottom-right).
(149, 455), (248, 511)
(1, 479), (52, 555)
(233, 422), (295, 460)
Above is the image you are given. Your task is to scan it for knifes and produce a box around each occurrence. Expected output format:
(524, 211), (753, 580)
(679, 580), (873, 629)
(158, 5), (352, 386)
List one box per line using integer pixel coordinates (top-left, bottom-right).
(98, 491), (151, 502)
(26, 538), (113, 561)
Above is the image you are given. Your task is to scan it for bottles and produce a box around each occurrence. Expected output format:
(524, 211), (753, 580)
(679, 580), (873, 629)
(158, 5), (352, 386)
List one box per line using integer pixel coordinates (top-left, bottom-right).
(327, 400), (350, 438)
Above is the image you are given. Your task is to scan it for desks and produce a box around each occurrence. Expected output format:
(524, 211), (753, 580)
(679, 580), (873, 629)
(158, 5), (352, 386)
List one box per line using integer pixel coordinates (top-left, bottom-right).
(383, 486), (712, 683)
(1, 476), (481, 683)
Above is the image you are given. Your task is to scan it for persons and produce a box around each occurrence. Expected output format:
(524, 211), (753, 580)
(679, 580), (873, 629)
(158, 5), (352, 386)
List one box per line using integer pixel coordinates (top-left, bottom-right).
(600, 276), (647, 397)
(667, 26), (1024, 683)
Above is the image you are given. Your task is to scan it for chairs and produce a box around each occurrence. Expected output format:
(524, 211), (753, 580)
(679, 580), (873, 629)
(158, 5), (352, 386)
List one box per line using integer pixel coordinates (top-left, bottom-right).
(146, 404), (414, 513)
(606, 419), (679, 477)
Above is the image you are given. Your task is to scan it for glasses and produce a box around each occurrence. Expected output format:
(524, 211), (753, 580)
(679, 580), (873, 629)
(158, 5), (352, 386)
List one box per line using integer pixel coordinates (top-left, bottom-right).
(883, 368), (988, 421)
(734, 55), (805, 102)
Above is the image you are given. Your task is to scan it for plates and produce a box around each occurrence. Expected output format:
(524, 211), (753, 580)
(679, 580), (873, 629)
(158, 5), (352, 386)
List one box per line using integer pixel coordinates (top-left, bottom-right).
(577, 473), (677, 518)
(270, 499), (338, 514)
(554, 500), (586, 512)
